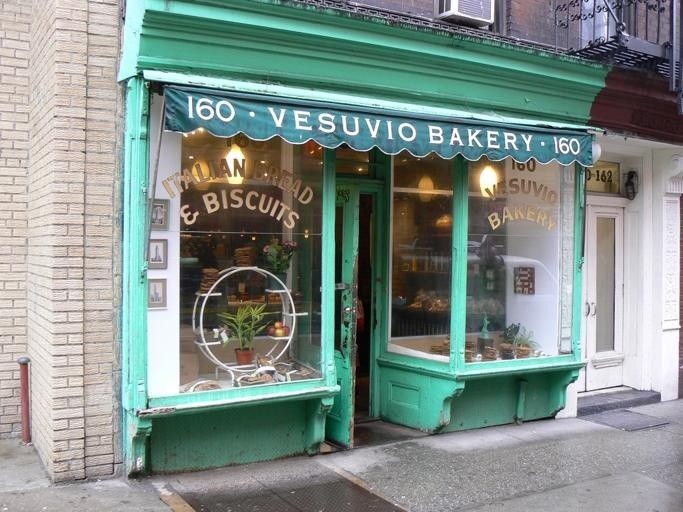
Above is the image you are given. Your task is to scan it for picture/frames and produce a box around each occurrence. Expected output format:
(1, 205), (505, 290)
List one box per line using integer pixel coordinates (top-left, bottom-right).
(148, 198), (170, 310)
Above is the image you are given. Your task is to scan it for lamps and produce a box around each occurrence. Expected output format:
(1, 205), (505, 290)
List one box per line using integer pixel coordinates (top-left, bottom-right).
(190, 137), (249, 187)
(591, 131), (605, 168)
(624, 171), (641, 204)
(478, 158), (499, 199)
(416, 162), (436, 203)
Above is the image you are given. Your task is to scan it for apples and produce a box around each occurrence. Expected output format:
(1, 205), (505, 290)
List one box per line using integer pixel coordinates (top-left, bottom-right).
(267, 322), (289, 336)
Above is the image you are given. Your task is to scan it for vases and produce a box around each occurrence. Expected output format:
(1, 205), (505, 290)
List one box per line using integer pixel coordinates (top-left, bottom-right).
(269, 272), (288, 290)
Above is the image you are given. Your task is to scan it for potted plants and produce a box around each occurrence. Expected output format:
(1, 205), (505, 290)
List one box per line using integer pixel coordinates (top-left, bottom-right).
(216, 299), (272, 366)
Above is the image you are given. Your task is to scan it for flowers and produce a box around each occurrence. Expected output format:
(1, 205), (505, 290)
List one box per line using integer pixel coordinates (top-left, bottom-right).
(254, 236), (300, 272)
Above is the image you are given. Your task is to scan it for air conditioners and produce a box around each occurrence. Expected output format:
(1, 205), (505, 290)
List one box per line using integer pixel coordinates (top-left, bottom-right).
(431, 1), (495, 28)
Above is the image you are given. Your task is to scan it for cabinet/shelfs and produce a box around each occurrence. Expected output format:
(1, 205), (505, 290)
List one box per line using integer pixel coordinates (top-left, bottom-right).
(193, 265), (307, 385)
